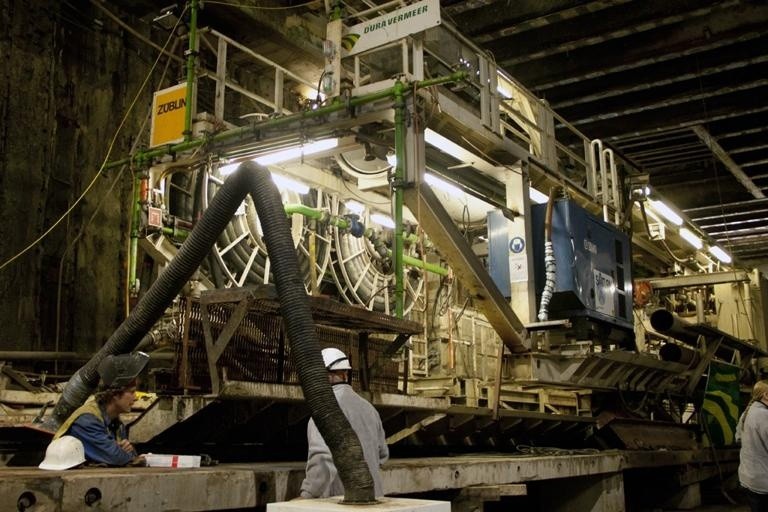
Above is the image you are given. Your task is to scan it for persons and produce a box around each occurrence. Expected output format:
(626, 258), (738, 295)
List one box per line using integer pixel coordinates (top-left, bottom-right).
(288, 347), (390, 505)
(42, 355), (146, 470)
(731, 378), (768, 512)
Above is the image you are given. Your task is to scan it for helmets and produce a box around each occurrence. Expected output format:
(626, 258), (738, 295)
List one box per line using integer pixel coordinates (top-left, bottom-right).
(322, 347), (351, 372)
(37, 435), (89, 471)
